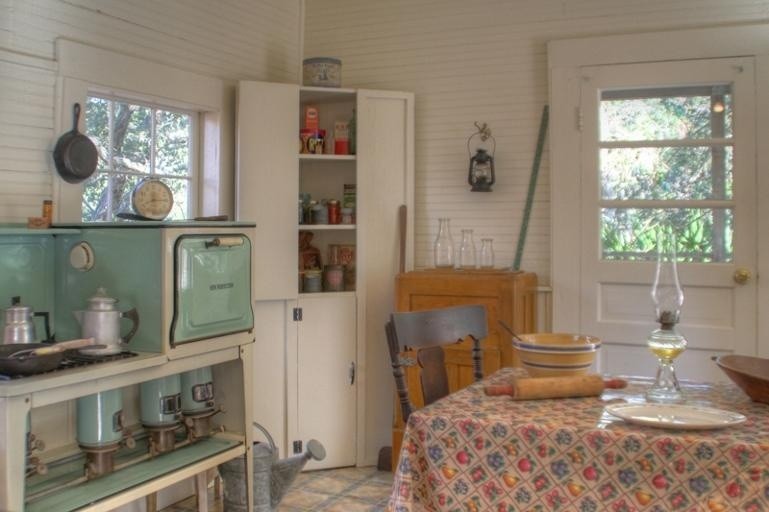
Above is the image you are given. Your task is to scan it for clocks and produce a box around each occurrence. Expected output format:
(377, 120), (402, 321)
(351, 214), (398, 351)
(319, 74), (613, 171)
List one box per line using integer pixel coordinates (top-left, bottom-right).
(132, 178), (174, 221)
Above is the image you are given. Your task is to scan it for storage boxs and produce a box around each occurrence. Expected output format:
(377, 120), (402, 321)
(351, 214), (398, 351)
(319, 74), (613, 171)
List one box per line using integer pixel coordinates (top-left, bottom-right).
(303, 57), (342, 87)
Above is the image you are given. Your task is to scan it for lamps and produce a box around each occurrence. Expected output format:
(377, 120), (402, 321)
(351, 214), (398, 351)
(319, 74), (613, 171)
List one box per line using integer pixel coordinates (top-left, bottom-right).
(468, 121), (497, 192)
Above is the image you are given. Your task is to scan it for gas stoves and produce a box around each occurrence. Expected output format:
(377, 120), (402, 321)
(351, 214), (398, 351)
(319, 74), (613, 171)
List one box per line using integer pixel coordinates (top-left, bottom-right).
(0, 350), (139, 382)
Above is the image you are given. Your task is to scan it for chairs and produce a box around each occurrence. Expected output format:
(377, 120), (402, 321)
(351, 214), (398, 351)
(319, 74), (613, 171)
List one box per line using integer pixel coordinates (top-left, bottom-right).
(385, 303), (489, 425)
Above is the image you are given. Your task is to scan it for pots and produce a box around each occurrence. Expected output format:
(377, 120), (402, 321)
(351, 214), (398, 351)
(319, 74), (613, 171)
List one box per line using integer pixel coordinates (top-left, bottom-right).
(54, 103), (98, 183)
(0, 344), (106, 374)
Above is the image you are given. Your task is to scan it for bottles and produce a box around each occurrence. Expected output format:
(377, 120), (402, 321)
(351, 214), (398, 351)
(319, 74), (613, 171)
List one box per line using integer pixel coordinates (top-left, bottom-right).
(460, 230), (477, 270)
(481, 240), (495, 271)
(298, 109), (356, 223)
(433, 218), (453, 269)
(43, 201), (51, 229)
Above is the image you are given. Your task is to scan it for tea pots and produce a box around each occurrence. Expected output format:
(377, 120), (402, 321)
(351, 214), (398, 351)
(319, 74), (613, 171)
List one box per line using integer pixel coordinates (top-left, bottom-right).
(0, 296), (53, 346)
(73, 288), (140, 356)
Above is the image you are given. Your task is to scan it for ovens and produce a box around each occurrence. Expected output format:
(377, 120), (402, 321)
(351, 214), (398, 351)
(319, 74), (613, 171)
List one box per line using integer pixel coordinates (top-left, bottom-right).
(54, 219), (257, 360)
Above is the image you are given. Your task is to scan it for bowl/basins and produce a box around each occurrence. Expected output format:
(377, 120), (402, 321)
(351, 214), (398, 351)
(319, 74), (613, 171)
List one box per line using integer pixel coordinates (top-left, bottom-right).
(716, 354), (769, 405)
(512, 334), (601, 379)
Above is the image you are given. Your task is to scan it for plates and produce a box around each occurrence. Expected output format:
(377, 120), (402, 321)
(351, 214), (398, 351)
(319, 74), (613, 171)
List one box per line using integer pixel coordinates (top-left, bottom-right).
(605, 402), (746, 432)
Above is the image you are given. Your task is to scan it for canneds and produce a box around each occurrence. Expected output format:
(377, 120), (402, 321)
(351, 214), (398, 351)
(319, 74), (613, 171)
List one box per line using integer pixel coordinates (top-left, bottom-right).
(327, 198), (342, 224)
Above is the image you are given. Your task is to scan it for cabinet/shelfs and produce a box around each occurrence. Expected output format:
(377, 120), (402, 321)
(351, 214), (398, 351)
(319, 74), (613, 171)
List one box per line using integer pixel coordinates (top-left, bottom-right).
(234, 80), (415, 472)
(0, 222), (257, 512)
(391, 266), (538, 475)
